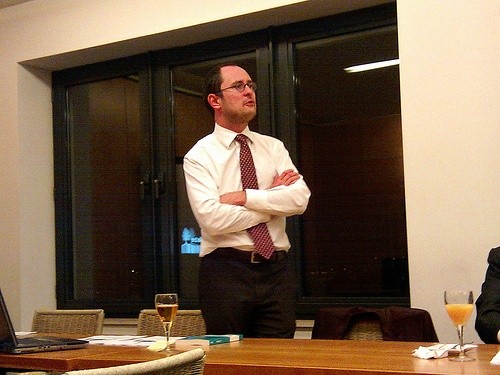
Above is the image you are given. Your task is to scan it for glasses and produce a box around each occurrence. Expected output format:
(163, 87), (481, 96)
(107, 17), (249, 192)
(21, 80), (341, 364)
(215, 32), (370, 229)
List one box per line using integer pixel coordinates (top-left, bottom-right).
(214, 81), (258, 92)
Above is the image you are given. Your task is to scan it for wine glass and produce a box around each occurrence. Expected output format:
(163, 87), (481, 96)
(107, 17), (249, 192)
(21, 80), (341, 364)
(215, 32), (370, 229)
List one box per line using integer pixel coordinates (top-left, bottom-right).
(155, 293), (179, 354)
(444, 290), (476, 363)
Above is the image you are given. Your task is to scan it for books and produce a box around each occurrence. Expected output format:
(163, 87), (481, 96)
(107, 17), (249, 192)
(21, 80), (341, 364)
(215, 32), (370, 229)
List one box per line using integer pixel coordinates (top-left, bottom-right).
(176, 335), (243, 346)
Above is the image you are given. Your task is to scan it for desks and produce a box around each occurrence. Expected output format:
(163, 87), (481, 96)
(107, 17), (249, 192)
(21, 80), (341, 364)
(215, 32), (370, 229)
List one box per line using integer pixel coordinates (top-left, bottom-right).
(0, 333), (500, 375)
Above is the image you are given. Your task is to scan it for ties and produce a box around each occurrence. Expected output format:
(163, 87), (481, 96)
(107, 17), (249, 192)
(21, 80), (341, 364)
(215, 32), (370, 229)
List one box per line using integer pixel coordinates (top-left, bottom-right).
(235, 134), (275, 260)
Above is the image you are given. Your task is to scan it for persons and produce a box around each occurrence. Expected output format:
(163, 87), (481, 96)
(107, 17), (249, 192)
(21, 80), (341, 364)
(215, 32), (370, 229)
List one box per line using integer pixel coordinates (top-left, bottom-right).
(183, 62), (311, 340)
(475, 246), (500, 345)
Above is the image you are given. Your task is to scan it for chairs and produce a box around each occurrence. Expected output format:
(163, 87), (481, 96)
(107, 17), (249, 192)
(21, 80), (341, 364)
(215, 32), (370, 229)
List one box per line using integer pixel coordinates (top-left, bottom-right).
(137, 309), (207, 337)
(32, 310), (105, 335)
(324, 306), (436, 342)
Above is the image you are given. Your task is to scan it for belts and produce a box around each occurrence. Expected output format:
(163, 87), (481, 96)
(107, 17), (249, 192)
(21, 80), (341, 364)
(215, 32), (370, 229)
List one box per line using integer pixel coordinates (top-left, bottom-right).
(216, 247), (286, 265)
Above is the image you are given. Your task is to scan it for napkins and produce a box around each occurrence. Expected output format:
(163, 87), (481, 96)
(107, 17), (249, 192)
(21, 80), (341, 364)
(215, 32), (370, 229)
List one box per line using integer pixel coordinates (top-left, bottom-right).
(411, 344), (478, 359)
